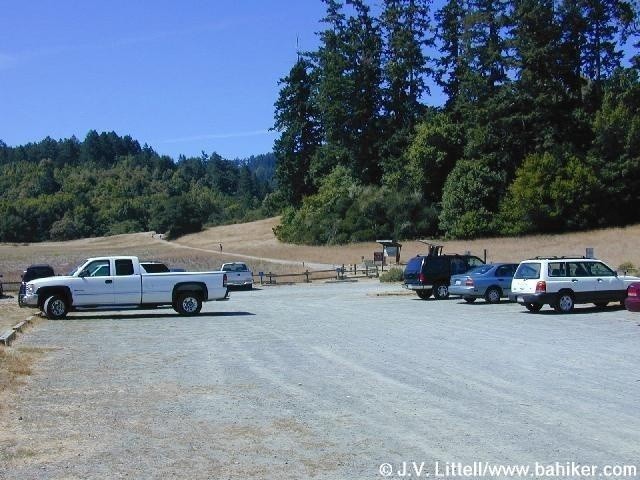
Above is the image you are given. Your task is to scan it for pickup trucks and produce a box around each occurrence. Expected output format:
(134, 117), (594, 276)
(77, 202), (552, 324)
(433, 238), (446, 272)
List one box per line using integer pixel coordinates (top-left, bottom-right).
(22, 255), (230, 320)
(216, 261), (254, 291)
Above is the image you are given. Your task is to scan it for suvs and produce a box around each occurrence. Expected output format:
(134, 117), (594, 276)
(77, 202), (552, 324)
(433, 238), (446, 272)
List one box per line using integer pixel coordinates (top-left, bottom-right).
(401, 253), (487, 300)
(508, 256), (640, 314)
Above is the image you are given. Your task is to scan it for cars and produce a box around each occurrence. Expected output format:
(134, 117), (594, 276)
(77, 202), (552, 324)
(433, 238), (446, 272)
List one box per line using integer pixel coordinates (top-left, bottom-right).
(448, 263), (537, 304)
(0, 274), (4, 296)
(168, 268), (186, 273)
(90, 260), (172, 276)
(624, 282), (640, 312)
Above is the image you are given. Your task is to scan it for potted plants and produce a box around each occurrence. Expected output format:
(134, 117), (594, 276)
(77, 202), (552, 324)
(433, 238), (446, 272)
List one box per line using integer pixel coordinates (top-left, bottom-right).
(375, 268), (417, 296)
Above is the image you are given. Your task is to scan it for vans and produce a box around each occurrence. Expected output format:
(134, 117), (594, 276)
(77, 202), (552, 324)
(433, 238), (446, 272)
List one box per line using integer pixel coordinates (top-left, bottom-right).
(19, 264), (59, 308)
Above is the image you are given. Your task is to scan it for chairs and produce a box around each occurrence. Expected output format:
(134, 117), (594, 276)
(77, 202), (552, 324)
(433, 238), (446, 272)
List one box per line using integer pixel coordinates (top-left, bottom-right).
(574, 267), (588, 276)
